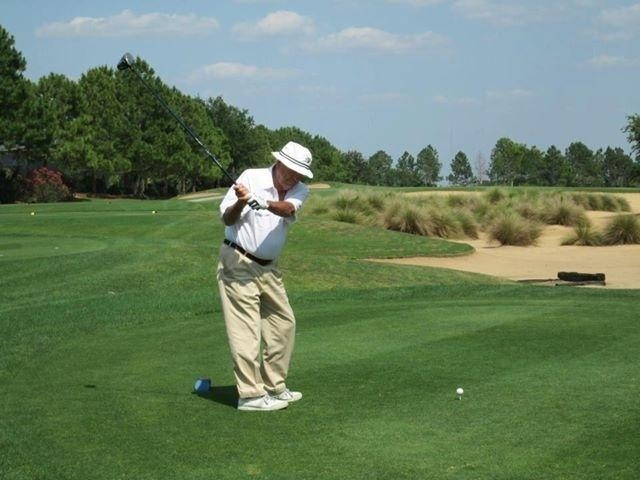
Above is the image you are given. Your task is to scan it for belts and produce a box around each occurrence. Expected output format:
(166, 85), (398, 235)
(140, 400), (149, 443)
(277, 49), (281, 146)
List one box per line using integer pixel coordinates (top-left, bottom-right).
(223, 238), (273, 266)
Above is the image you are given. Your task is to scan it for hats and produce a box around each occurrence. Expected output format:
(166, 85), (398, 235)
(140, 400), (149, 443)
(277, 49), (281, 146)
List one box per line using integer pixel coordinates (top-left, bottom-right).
(271, 140), (314, 179)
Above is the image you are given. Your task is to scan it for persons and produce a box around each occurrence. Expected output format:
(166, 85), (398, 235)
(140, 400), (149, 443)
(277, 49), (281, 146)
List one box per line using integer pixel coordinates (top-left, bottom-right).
(215, 139), (316, 415)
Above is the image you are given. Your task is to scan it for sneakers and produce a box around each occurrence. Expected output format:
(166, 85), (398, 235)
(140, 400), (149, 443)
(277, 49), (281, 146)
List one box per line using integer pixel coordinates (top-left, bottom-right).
(237, 392), (289, 412)
(270, 388), (303, 402)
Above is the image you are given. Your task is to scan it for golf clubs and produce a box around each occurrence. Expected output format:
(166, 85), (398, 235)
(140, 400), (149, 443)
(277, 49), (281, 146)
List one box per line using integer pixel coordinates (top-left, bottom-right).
(117, 53), (260, 210)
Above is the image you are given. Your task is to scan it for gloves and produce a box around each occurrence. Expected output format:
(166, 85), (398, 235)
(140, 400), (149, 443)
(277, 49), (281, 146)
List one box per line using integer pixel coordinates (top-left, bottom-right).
(246, 193), (269, 211)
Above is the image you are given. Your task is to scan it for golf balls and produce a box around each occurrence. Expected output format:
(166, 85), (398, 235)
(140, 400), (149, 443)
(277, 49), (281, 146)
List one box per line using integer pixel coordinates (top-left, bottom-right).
(151, 211), (155, 214)
(30, 211), (35, 216)
(457, 387), (463, 394)
